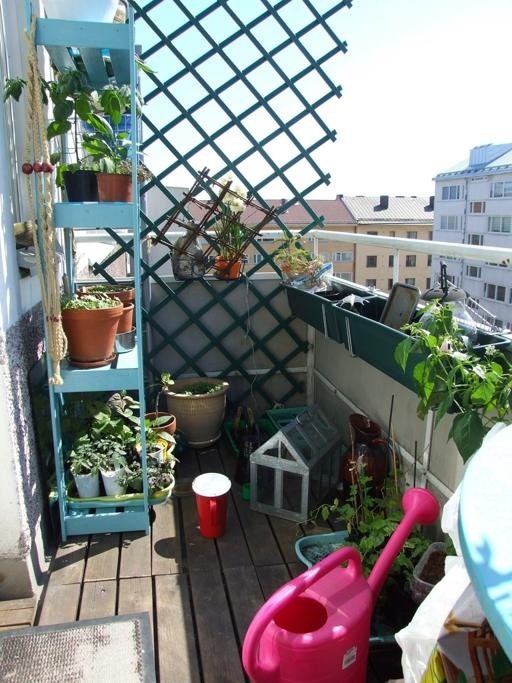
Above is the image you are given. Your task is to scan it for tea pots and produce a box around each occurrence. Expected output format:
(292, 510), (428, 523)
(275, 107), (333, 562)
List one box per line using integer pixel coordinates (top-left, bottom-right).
(340, 411), (402, 513)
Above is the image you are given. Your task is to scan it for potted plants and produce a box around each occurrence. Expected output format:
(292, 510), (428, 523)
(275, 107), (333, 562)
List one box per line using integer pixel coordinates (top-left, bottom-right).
(80, 80), (146, 202)
(1, 61), (99, 204)
(64, 369), (234, 509)
(59, 282), (137, 368)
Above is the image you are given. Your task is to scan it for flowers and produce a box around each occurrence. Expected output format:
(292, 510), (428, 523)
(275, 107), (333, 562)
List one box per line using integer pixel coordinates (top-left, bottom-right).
(210, 176), (250, 256)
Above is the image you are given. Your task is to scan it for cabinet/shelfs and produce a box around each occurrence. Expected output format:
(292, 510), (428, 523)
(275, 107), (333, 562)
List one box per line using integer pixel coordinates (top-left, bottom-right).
(21, 0), (160, 546)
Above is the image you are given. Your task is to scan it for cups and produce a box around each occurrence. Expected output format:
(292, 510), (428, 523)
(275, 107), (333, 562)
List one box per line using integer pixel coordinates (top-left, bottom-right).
(191, 470), (231, 539)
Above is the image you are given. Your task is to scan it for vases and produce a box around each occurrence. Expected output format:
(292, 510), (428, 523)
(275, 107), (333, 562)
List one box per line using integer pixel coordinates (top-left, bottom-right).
(213, 254), (244, 281)
(282, 275), (380, 345)
(331, 294), (512, 414)
(410, 541), (450, 604)
(294, 528), (352, 570)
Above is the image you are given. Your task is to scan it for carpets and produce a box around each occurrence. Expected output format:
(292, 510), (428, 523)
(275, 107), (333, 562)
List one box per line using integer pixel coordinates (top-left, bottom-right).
(0, 609), (162, 682)
